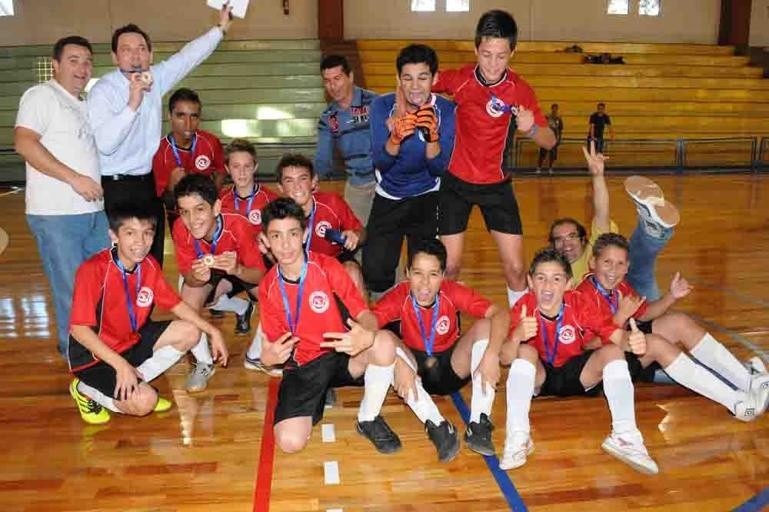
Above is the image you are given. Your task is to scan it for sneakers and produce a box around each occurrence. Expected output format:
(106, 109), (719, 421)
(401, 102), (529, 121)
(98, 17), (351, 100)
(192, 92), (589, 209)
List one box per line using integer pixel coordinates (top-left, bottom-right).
(498, 430), (535, 471)
(154, 396), (172, 413)
(68, 378), (111, 425)
(601, 428), (659, 476)
(423, 413), (462, 464)
(727, 356), (769, 423)
(623, 176), (681, 239)
(463, 413), (495, 456)
(186, 352), (216, 393)
(355, 414), (401, 454)
(234, 297), (256, 337)
(243, 349), (285, 378)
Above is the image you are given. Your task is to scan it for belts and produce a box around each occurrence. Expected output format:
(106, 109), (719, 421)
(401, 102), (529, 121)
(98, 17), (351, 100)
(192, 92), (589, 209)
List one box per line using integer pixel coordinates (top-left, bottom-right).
(102, 172), (154, 183)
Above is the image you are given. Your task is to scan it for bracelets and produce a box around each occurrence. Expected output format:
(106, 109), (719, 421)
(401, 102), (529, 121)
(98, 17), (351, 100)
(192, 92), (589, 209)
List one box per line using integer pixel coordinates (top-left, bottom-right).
(528, 124), (538, 137)
(216, 23), (230, 37)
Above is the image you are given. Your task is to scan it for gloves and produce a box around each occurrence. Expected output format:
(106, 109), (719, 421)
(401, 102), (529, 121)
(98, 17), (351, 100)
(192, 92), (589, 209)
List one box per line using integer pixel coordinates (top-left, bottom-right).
(390, 104), (441, 145)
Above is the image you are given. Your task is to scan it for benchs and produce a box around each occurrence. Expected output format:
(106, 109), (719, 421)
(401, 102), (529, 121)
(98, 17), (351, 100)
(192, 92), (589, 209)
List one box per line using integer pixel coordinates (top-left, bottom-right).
(357, 36), (768, 170)
(1, 36), (333, 147)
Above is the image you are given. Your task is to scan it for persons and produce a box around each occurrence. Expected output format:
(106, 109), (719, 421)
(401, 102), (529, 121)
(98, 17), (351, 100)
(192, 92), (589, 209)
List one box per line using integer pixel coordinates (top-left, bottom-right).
(304, 50), (402, 301)
(216, 141), (281, 334)
(546, 137), (767, 398)
(388, 9), (559, 319)
(151, 87), (228, 312)
(582, 102), (614, 157)
(497, 246), (662, 473)
(569, 232), (767, 427)
(535, 104), (563, 177)
(167, 177), (284, 393)
(61, 200), (230, 423)
(274, 157), (366, 296)
(361, 43), (457, 305)
(256, 196), (402, 455)
(88, 1), (237, 343)
(368, 238), (510, 463)
(11, 37), (113, 366)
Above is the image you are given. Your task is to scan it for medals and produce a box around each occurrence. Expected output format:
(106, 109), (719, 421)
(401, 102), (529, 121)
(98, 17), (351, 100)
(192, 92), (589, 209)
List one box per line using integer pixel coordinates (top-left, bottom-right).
(129, 332), (142, 343)
(486, 99), (507, 118)
(131, 71), (142, 83)
(426, 356), (438, 368)
(202, 254), (214, 266)
(141, 70), (154, 85)
